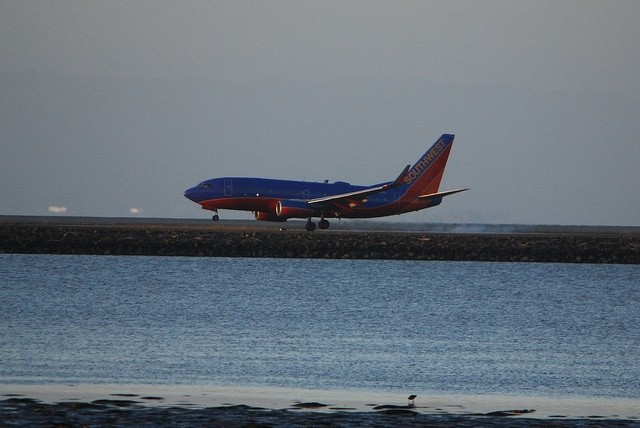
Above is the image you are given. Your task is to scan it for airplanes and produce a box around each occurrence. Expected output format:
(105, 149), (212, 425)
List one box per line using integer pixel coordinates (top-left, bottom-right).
(184, 133), (471, 231)
